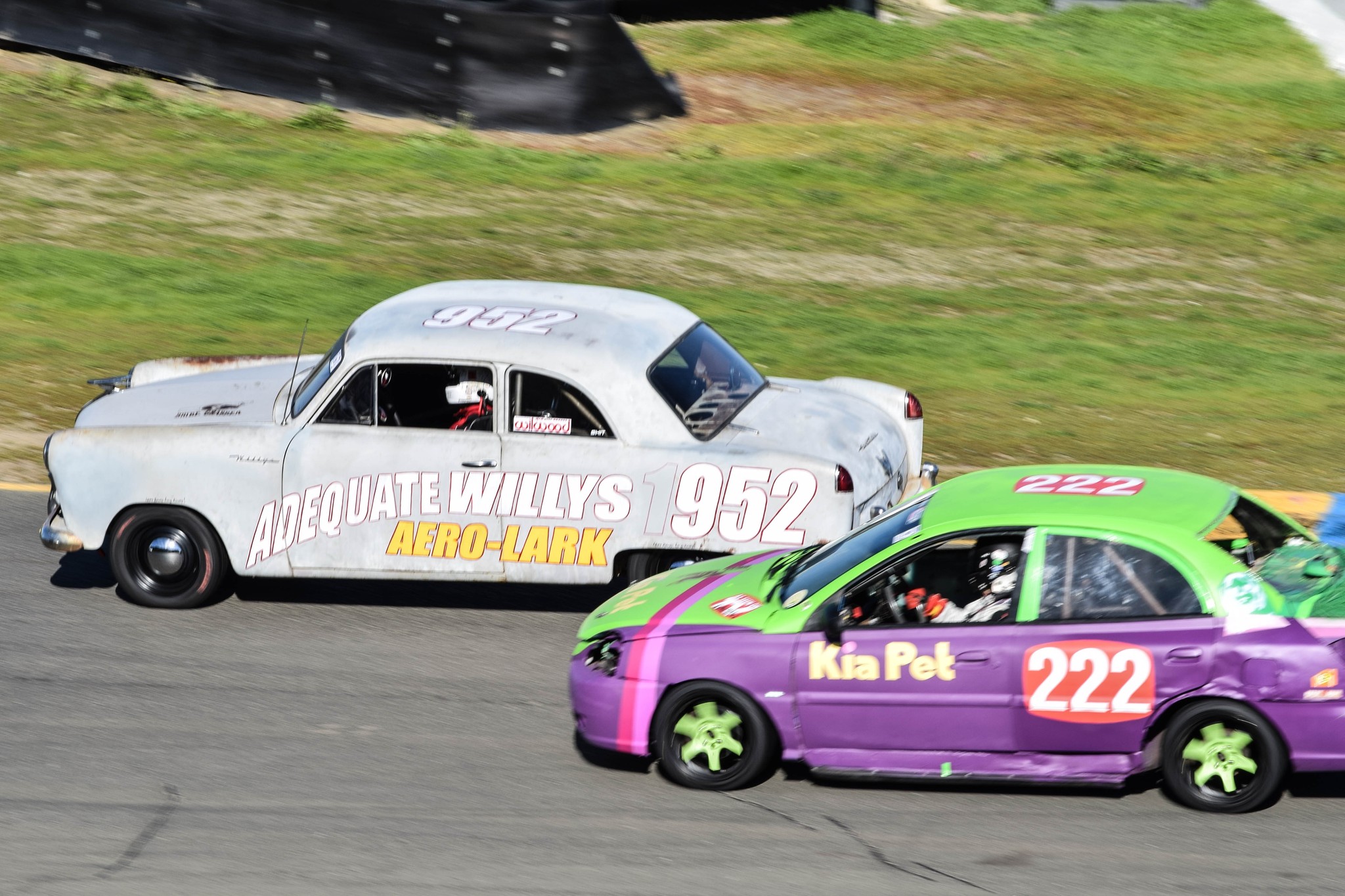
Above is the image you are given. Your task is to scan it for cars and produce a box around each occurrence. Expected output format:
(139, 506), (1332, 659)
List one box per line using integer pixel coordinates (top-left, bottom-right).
(566, 462), (1345, 816)
(38, 275), (939, 608)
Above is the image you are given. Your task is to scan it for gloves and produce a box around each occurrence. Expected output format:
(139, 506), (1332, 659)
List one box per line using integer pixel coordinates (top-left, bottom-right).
(905, 588), (926, 609)
(924, 594), (948, 619)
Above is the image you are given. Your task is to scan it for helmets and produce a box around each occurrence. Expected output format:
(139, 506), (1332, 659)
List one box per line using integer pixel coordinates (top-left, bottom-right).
(968, 536), (1023, 594)
(445, 366), (493, 404)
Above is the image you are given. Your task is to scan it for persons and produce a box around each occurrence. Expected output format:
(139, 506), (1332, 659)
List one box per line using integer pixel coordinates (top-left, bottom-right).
(445, 369), (494, 430)
(905, 537), (1021, 622)
